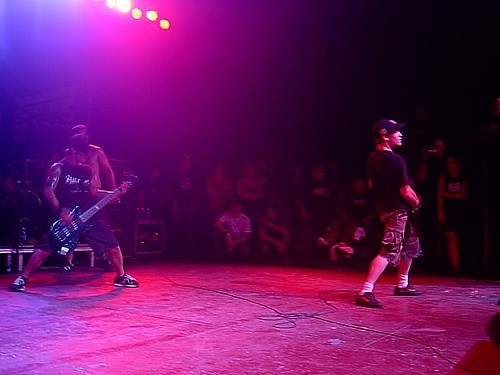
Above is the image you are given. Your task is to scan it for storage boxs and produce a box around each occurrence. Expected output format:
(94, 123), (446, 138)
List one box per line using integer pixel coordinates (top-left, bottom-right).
(132, 219), (166, 254)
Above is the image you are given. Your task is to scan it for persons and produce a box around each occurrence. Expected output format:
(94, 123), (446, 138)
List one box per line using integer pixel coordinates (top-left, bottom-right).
(356, 118), (423, 307)
(62, 125), (116, 272)
(161, 140), (471, 280)
(9, 135), (139, 293)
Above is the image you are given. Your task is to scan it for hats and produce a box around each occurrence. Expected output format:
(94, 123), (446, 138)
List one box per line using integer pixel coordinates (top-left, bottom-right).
(371, 119), (406, 138)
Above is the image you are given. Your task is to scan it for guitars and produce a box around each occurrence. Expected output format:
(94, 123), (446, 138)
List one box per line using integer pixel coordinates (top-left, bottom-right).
(47, 170), (138, 251)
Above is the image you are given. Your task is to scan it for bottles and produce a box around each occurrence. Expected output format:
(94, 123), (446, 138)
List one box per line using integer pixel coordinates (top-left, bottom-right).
(135, 207), (152, 220)
(19, 227), (26, 246)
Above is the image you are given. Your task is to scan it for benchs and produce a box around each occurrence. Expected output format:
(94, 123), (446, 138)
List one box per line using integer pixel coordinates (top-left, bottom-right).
(0, 244), (95, 272)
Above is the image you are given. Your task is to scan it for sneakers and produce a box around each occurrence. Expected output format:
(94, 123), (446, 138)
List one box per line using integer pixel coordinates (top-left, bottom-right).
(114, 274), (139, 288)
(64, 265), (74, 271)
(356, 292), (384, 307)
(10, 275), (26, 292)
(394, 286), (421, 296)
